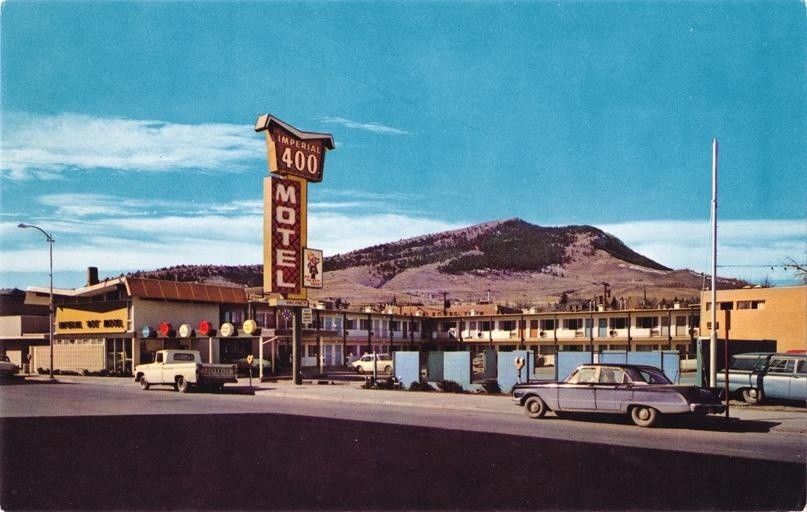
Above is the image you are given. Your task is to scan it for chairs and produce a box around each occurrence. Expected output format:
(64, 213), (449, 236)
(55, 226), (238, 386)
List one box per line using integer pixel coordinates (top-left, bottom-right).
(605, 371), (617, 383)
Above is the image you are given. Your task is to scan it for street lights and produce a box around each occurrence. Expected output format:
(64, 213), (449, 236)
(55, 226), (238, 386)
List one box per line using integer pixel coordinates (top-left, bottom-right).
(18, 223), (54, 376)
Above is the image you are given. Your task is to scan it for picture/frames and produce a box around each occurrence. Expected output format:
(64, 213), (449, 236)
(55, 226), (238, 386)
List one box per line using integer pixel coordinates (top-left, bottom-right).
(302, 247), (323, 289)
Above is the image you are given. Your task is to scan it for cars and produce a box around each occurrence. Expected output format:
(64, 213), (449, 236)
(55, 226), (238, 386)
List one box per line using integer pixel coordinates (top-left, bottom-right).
(353, 353), (393, 375)
(512, 364), (725, 428)
(0, 356), (16, 374)
(719, 352), (807, 405)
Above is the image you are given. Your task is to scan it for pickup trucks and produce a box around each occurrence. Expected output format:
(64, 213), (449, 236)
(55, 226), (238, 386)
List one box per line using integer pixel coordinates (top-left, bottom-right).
(135, 349), (238, 392)
